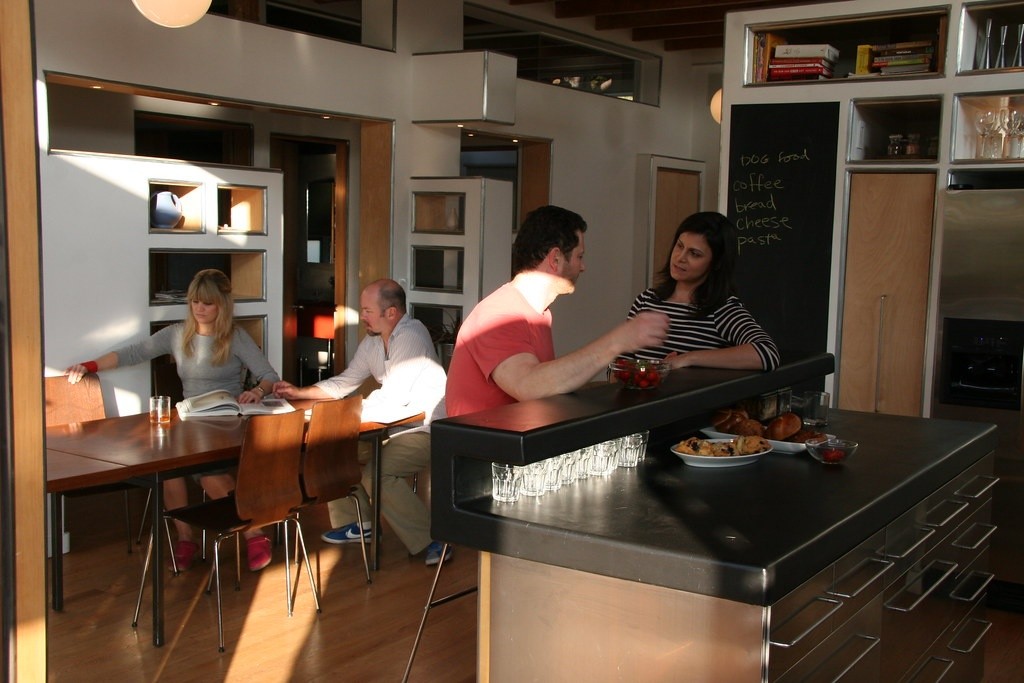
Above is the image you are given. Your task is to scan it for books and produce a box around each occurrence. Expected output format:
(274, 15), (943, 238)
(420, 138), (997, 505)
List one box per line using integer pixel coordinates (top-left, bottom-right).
(753, 31), (840, 83)
(848, 17), (947, 78)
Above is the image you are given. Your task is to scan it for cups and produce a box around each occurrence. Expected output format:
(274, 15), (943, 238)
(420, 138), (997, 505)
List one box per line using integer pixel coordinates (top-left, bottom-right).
(149, 396), (171, 422)
(491, 430), (650, 502)
(802, 391), (830, 427)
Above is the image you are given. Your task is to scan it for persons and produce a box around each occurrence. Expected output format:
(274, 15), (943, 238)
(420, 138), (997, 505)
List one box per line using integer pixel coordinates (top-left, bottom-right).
(615, 211), (780, 370)
(446, 204), (671, 418)
(273, 278), (454, 566)
(62, 270), (281, 572)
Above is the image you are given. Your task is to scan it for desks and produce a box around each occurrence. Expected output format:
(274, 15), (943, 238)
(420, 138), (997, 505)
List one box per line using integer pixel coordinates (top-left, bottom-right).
(45, 388), (426, 616)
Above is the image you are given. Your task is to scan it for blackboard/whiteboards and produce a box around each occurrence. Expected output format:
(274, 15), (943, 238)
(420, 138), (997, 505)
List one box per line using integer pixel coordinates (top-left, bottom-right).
(725, 100), (840, 406)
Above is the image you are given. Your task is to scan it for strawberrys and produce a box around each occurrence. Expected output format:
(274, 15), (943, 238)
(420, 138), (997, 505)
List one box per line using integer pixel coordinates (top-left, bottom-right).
(614, 358), (661, 388)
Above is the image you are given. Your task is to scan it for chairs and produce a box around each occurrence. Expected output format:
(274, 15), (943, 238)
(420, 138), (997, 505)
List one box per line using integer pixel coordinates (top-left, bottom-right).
(161, 409), (320, 653)
(275, 391), (371, 608)
(150, 358), (254, 573)
(44, 374), (138, 555)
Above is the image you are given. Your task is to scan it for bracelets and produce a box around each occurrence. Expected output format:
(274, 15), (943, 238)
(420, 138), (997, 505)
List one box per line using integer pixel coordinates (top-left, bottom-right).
(79, 361), (98, 373)
(255, 386), (265, 396)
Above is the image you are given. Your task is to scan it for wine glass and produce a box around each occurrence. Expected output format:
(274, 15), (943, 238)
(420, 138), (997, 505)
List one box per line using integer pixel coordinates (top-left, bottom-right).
(972, 107), (1023, 159)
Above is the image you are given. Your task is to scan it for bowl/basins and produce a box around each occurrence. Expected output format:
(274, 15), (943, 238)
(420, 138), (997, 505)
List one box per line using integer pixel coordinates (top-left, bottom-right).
(805, 438), (859, 464)
(608, 359), (672, 391)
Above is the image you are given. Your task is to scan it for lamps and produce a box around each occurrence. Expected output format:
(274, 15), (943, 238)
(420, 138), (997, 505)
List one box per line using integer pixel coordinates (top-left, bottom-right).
(131, 0), (218, 32)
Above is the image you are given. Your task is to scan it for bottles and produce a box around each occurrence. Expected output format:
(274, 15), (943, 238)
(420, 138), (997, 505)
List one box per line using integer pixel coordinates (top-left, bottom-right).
(888, 132), (938, 157)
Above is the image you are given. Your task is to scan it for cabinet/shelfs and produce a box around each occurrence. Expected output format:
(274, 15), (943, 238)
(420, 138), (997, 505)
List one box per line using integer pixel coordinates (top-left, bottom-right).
(146, 179), (269, 362)
(719, 0), (1024, 175)
(406, 176), (515, 339)
(490, 448), (1024, 683)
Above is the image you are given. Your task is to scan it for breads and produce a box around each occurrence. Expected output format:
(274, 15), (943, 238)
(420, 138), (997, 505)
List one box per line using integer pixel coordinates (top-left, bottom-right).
(712, 406), (829, 444)
(674, 434), (770, 457)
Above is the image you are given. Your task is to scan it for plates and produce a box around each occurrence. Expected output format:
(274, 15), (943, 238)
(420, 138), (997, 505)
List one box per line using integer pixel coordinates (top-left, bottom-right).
(699, 427), (836, 455)
(670, 438), (773, 468)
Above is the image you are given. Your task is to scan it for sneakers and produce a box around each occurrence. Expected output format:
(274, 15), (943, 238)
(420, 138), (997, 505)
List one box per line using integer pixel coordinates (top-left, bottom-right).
(320, 521), (383, 544)
(170, 541), (198, 571)
(425, 541), (453, 564)
(246, 532), (272, 571)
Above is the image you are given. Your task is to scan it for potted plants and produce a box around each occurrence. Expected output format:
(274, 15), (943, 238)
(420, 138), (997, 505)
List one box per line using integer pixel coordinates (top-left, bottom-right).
(417, 308), (464, 373)
